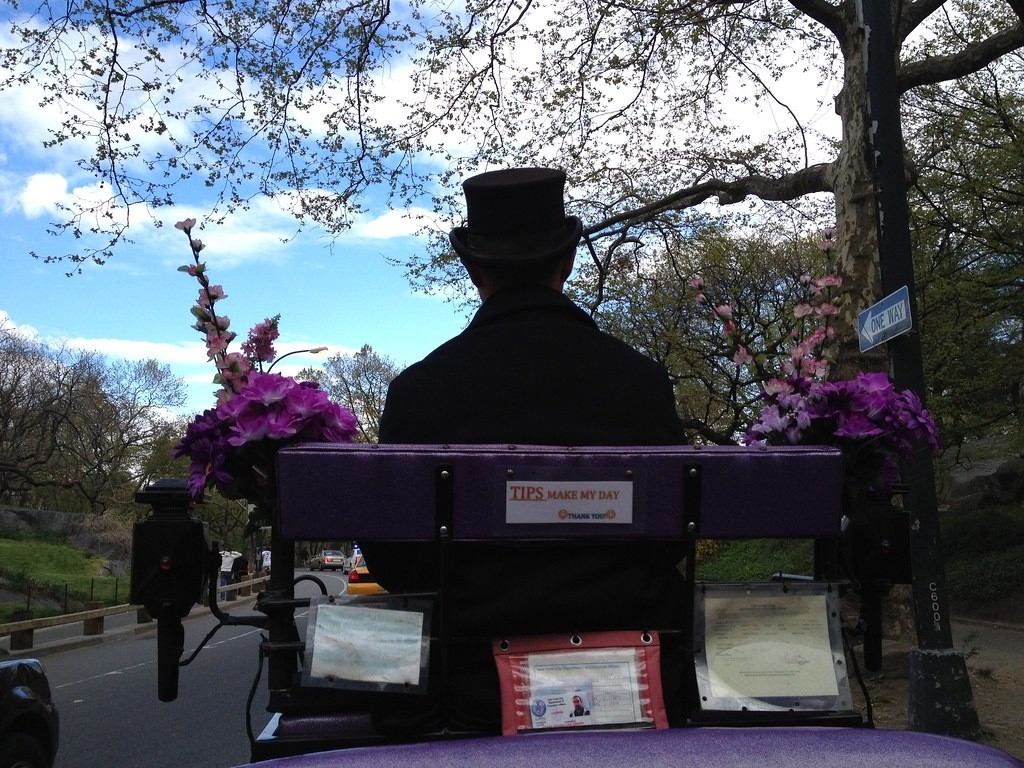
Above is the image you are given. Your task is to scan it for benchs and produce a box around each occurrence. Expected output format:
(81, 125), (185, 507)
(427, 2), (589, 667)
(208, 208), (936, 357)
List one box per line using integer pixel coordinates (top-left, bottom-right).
(262, 440), (863, 747)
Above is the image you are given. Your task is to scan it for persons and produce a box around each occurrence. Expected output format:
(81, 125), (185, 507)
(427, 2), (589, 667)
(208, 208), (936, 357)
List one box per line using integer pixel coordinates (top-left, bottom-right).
(296, 547), (309, 567)
(219, 550), (242, 600)
(569, 696), (590, 718)
(199, 552), (249, 607)
(356, 168), (690, 636)
(262, 546), (271, 567)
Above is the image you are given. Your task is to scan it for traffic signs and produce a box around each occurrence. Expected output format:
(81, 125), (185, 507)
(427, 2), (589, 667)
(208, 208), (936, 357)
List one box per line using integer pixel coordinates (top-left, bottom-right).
(857, 285), (913, 354)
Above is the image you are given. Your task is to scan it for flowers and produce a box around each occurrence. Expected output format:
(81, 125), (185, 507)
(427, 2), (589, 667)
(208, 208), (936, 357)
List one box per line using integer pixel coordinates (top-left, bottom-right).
(689, 230), (940, 487)
(170, 217), (362, 499)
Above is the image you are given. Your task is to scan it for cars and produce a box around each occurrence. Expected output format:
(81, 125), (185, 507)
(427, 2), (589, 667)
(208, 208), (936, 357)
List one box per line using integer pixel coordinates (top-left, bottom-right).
(347, 555), (390, 595)
(309, 549), (345, 571)
(343, 544), (367, 574)
(0, 658), (60, 768)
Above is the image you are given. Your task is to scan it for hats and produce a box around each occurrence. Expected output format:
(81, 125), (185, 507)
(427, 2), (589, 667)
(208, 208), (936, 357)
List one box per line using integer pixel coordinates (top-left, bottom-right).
(450, 168), (583, 267)
